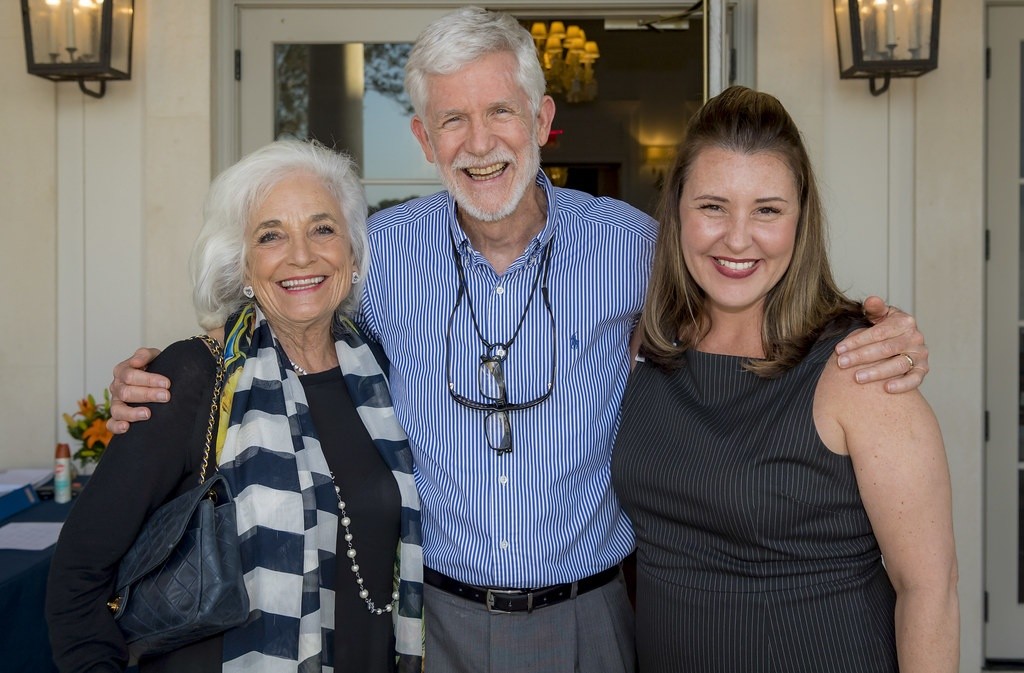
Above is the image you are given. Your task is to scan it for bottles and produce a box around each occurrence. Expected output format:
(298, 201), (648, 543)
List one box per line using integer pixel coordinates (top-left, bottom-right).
(54, 443), (72, 504)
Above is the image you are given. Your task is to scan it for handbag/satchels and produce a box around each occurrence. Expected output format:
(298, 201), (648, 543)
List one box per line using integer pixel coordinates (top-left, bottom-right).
(104, 333), (250, 662)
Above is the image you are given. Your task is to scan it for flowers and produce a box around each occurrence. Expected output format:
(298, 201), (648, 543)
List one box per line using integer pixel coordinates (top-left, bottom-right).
(62, 388), (113, 468)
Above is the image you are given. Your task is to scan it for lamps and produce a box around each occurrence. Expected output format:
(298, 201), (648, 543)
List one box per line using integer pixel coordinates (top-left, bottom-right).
(833, 0), (942, 96)
(20, 0), (135, 98)
(531, 22), (599, 103)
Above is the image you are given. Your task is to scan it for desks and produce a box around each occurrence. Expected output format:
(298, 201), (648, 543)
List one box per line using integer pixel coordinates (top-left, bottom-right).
(0, 475), (94, 673)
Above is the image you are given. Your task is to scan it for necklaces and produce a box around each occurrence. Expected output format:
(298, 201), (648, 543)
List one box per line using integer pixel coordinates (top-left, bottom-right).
(287, 353), (403, 615)
(446, 216), (546, 361)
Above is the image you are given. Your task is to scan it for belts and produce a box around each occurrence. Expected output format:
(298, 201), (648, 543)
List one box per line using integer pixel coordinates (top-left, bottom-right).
(423, 562), (621, 616)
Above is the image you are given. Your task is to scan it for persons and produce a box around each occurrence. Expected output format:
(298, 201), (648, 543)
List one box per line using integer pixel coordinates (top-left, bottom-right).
(48, 136), (428, 673)
(610, 85), (967, 673)
(104, 6), (931, 673)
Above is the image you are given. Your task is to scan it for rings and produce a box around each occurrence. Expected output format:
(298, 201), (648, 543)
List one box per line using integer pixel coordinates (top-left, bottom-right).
(900, 352), (914, 370)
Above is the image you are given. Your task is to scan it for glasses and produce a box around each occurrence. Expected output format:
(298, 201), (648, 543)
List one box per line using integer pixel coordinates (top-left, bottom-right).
(478, 353), (513, 457)
(446, 278), (557, 413)
